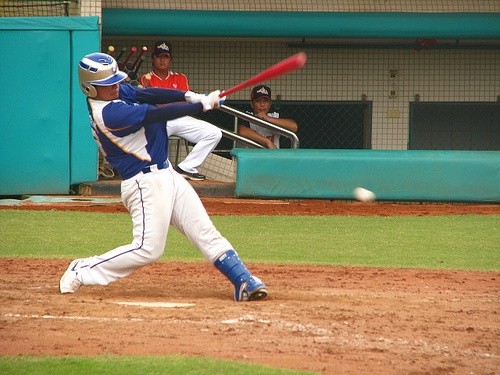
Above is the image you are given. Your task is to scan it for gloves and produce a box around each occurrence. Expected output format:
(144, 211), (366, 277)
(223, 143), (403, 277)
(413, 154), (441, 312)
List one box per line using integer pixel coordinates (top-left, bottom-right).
(185, 89), (227, 112)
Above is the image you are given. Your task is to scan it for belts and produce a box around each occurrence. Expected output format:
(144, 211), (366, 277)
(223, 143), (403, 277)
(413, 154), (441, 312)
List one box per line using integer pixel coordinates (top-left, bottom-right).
(142, 161), (169, 174)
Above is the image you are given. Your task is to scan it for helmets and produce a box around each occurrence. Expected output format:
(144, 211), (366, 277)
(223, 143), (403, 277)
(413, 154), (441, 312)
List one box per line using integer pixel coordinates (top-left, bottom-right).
(78, 53), (128, 98)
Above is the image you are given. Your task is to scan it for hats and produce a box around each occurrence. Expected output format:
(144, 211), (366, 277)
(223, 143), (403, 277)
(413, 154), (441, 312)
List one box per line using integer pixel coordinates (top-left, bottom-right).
(251, 85), (272, 101)
(152, 39), (173, 56)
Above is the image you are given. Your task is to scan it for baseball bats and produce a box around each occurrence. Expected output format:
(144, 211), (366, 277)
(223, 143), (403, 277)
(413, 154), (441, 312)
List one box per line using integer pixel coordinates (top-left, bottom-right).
(107, 45), (147, 88)
(218, 51), (307, 99)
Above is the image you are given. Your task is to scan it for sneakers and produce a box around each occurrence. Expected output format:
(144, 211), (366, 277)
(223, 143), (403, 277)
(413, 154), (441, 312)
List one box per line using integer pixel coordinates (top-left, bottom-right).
(175, 166), (206, 181)
(235, 277), (268, 302)
(58, 258), (82, 294)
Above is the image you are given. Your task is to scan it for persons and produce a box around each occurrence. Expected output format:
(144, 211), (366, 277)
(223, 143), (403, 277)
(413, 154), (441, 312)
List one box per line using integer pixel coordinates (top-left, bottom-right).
(59, 53), (267, 301)
(239, 84), (298, 149)
(137, 40), (222, 180)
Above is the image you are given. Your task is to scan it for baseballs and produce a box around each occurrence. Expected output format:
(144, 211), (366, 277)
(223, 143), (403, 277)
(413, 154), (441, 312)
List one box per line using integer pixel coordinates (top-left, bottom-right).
(353, 187), (376, 203)
